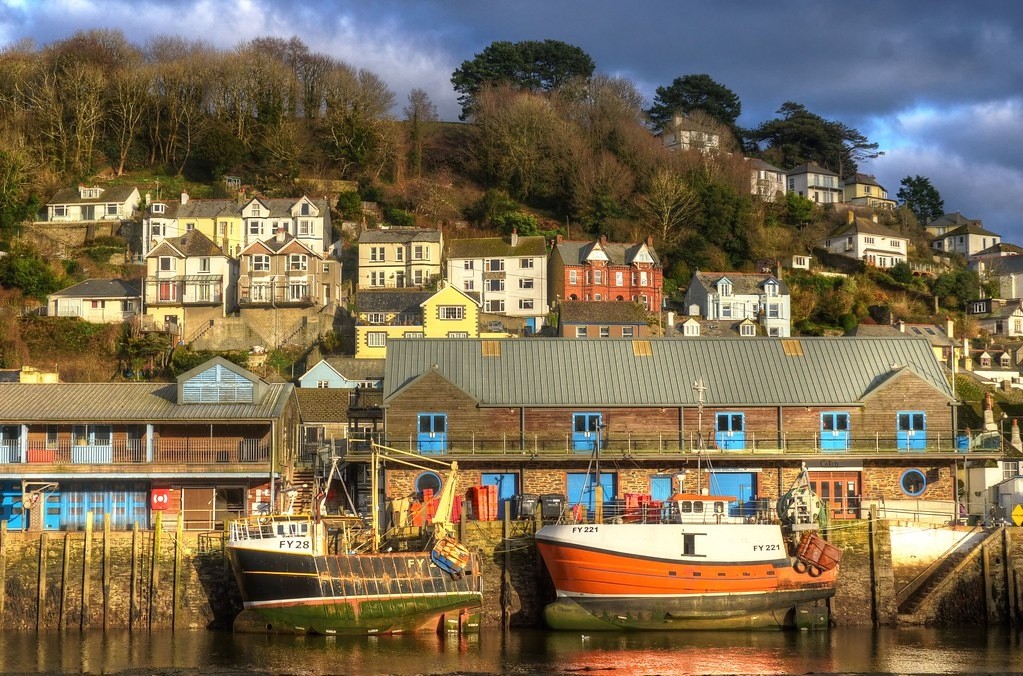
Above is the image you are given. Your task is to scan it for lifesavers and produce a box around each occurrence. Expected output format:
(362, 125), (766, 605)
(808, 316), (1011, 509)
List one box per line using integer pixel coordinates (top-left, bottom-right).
(794, 560), (810, 573)
(809, 565), (822, 577)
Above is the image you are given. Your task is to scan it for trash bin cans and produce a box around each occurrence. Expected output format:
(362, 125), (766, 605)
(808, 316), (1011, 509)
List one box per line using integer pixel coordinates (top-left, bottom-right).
(540, 493), (566, 519)
(514, 493), (539, 518)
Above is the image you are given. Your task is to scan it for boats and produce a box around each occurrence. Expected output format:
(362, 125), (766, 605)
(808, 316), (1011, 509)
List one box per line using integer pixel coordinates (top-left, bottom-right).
(222, 412), (486, 634)
(533, 374), (844, 630)
